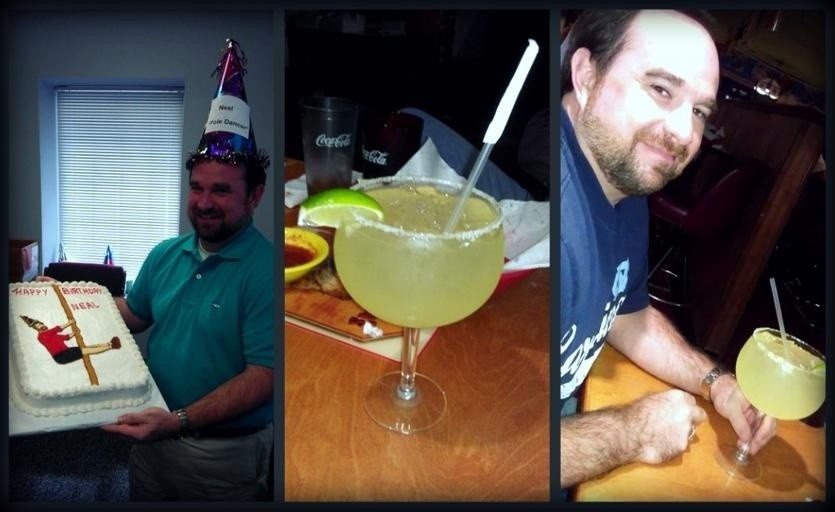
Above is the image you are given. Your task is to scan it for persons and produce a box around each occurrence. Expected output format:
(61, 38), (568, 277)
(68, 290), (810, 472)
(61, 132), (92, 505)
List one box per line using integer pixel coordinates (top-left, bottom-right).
(33, 158), (276, 501)
(560, 8), (777, 502)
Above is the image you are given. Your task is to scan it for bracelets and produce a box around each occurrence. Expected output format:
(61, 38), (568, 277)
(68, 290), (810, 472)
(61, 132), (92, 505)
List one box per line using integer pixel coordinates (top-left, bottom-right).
(700, 366), (735, 403)
(175, 407), (190, 441)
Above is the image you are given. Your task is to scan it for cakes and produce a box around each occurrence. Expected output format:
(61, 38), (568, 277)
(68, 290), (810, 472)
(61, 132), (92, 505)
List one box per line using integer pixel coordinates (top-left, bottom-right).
(8, 279), (153, 418)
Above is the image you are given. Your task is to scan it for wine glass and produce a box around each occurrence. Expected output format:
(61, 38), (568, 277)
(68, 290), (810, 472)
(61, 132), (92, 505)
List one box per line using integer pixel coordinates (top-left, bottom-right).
(716, 327), (827, 481)
(333, 176), (506, 436)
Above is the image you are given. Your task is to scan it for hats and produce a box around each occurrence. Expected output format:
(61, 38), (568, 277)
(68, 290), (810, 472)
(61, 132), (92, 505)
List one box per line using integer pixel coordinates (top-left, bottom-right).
(186, 38), (271, 170)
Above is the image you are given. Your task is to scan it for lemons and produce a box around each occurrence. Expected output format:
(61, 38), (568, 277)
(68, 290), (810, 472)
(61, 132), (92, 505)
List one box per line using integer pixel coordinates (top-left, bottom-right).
(296, 189), (386, 228)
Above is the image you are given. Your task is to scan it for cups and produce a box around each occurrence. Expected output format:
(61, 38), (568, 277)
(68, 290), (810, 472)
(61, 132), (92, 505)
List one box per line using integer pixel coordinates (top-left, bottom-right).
(384, 114), (424, 165)
(299, 90), (360, 196)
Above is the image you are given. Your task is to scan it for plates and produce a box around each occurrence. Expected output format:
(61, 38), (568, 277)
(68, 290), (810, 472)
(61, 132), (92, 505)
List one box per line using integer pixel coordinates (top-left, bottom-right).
(284, 225), (329, 286)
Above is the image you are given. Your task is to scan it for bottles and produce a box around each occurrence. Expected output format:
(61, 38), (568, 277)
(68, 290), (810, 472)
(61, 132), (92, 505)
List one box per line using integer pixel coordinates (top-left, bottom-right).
(104, 246), (112, 265)
